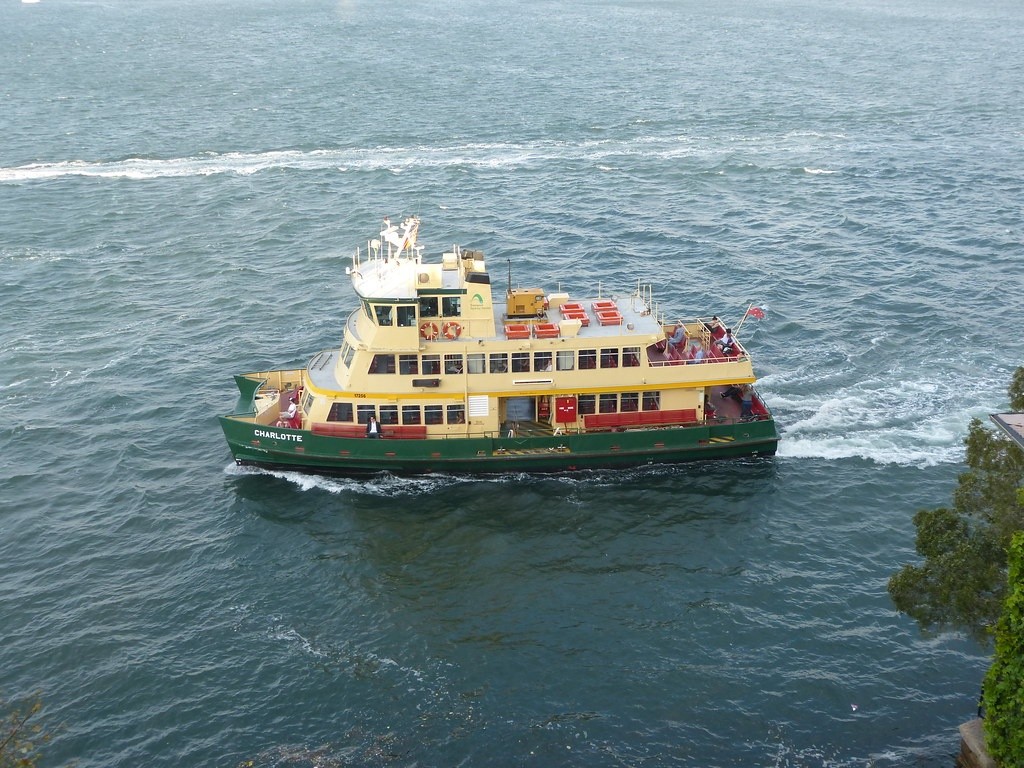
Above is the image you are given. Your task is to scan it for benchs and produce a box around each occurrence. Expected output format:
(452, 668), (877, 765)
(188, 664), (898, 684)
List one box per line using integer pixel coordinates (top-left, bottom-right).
(584, 408), (697, 435)
(656, 327), (742, 364)
(286, 411), (301, 429)
(704, 401), (716, 416)
(311, 423), (427, 438)
(289, 386), (303, 401)
(737, 388), (769, 421)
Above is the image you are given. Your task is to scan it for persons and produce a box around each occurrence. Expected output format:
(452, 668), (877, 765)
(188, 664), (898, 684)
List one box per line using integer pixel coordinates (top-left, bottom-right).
(699, 315), (719, 333)
(452, 355), (555, 374)
(713, 328), (735, 351)
(364, 416), (384, 439)
(704, 384), (756, 421)
(686, 345), (704, 364)
(278, 397), (297, 422)
(668, 322), (685, 348)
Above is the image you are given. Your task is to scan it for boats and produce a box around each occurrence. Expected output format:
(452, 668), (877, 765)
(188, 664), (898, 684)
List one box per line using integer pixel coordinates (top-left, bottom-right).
(217, 215), (782, 479)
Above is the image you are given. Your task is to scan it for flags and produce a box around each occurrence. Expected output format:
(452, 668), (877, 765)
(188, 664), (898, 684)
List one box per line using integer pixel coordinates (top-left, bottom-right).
(747, 307), (765, 319)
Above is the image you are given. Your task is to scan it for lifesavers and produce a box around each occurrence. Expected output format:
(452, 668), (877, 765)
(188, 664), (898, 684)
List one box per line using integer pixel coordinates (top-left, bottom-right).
(442, 322), (462, 340)
(419, 321), (439, 340)
